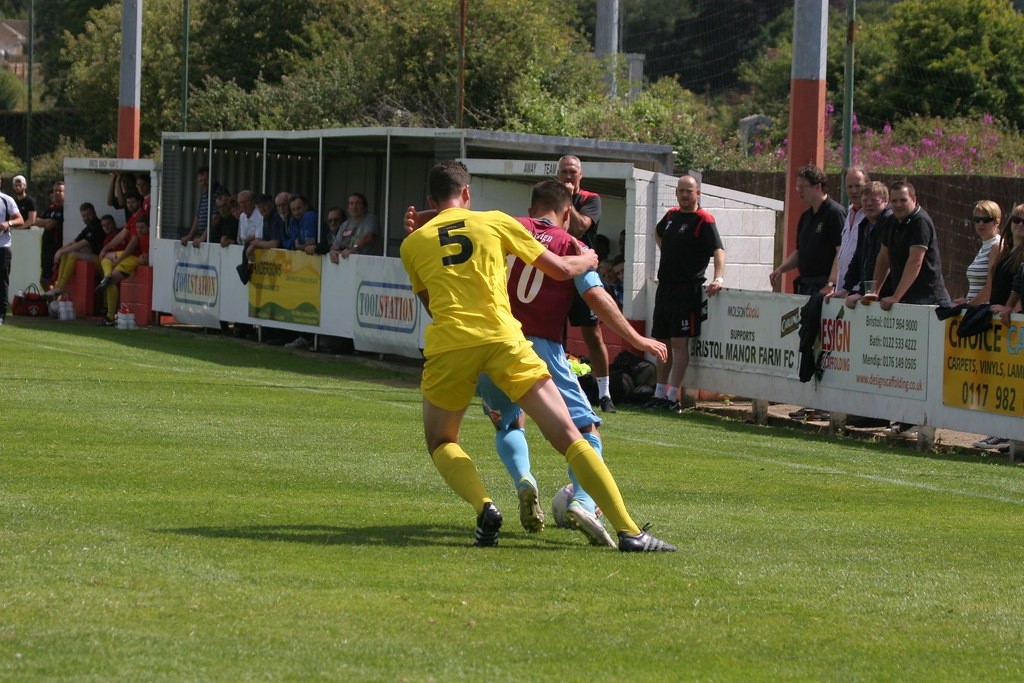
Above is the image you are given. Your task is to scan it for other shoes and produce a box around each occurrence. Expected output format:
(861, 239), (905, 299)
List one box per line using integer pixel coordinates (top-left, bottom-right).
(0, 316), (7, 324)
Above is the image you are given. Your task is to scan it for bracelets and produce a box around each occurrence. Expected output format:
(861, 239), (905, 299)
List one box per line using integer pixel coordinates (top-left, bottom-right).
(9, 220), (13, 227)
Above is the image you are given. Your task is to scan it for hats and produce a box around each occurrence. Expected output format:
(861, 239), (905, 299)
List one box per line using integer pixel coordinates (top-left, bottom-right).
(12, 175), (27, 184)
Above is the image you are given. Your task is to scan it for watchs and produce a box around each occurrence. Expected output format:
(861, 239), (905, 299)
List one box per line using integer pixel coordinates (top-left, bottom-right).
(826, 282), (836, 287)
(353, 244), (360, 251)
(714, 277), (724, 284)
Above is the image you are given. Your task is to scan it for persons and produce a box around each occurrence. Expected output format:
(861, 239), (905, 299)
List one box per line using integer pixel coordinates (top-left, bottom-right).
(94, 175), (151, 326)
(0, 191), (24, 325)
(405, 180), (668, 546)
(769, 166), (952, 439)
(180, 167), (382, 353)
(595, 230), (625, 311)
(641, 174), (726, 411)
(36, 181), (64, 293)
(42, 202), (107, 297)
(10, 175), (37, 229)
(952, 200), (1024, 453)
(556, 155), (617, 414)
(400, 162), (681, 553)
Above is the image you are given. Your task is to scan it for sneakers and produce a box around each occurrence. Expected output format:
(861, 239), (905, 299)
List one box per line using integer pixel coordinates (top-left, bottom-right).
(666, 394), (679, 412)
(883, 422), (918, 438)
(598, 396), (616, 413)
(998, 446), (1010, 453)
(94, 317), (114, 326)
(474, 502), (503, 547)
(644, 394), (664, 411)
(94, 276), (112, 291)
(518, 477), (544, 532)
(973, 436), (1009, 449)
(789, 408), (830, 420)
(284, 336), (309, 351)
(565, 500), (617, 548)
(617, 523), (678, 552)
(311, 339), (330, 351)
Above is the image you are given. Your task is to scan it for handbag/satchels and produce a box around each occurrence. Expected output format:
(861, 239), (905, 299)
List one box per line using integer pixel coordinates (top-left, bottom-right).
(12, 282), (49, 317)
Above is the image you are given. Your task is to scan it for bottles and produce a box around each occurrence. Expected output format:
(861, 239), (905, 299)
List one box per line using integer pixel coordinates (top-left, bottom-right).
(16, 289), (24, 298)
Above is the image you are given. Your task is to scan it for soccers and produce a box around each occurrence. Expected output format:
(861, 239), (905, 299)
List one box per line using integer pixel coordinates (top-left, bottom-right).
(552, 482), (603, 529)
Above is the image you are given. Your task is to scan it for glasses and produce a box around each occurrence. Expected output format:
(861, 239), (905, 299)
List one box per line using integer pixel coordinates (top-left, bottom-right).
(973, 216), (996, 223)
(1012, 216), (1024, 225)
(796, 184), (812, 192)
